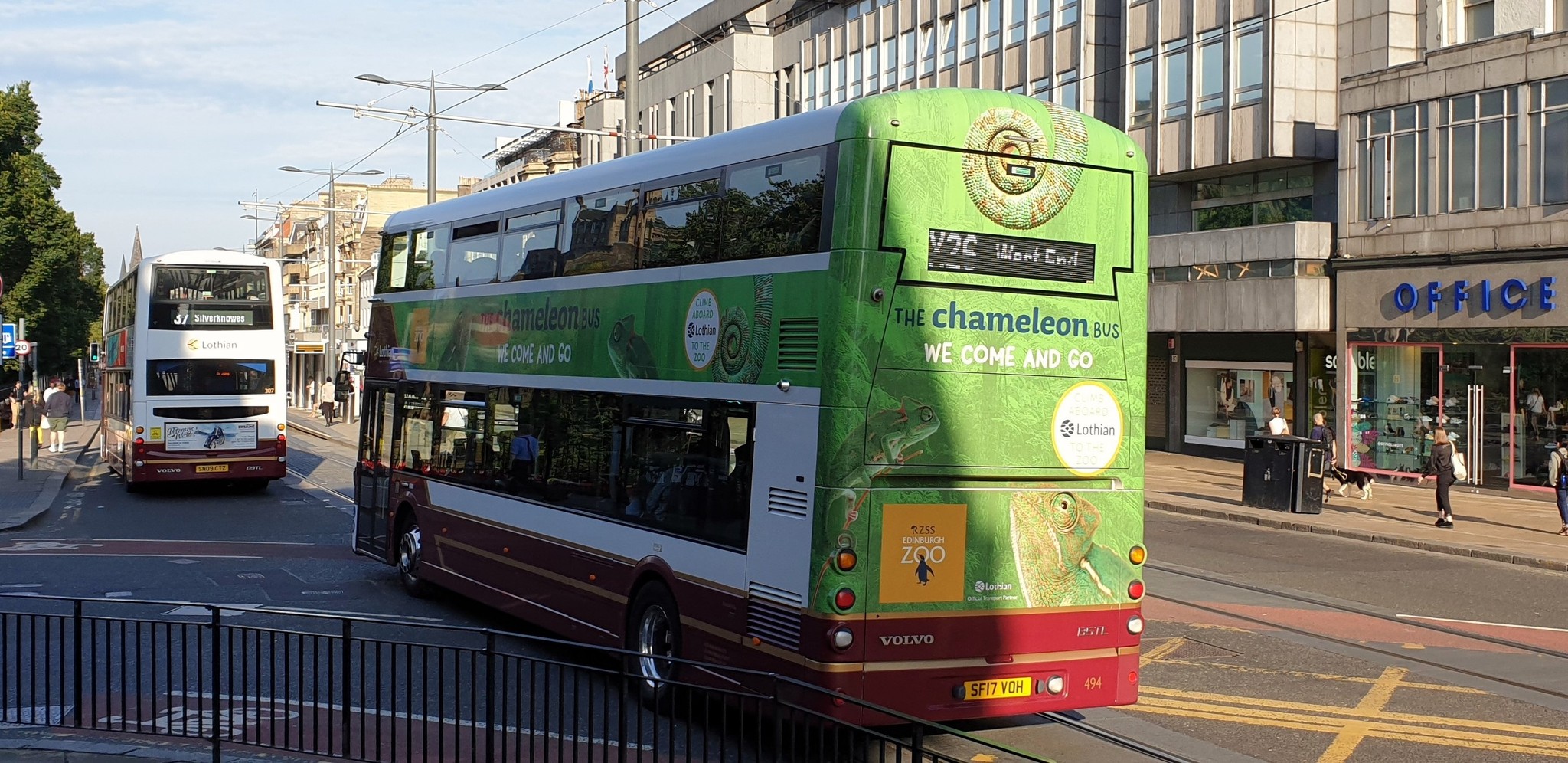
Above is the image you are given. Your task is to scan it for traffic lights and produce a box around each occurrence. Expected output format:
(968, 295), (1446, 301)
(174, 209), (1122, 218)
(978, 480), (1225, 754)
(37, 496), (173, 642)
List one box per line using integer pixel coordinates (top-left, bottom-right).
(89, 343), (99, 362)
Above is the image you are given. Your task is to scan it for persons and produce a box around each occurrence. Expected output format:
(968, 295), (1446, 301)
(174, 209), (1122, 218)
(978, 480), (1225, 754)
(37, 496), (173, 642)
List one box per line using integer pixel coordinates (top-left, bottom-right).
(1269, 407), (1290, 435)
(507, 424), (539, 498)
(306, 376), (315, 404)
(1518, 379), (1564, 441)
(307, 401), (339, 418)
(1309, 413), (1337, 503)
(1220, 376), (1234, 419)
(1548, 435), (1568, 536)
(321, 376), (335, 427)
(75, 374), (85, 404)
(8, 373), (74, 453)
(1268, 384), (1276, 407)
(1417, 427), (1457, 529)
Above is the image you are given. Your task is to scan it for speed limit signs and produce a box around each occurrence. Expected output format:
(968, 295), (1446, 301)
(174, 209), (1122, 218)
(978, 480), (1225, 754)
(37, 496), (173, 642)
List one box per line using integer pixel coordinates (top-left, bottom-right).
(15, 339), (32, 355)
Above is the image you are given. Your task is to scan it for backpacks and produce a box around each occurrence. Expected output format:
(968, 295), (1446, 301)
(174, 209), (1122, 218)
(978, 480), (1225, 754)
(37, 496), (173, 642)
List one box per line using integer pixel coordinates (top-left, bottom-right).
(1316, 425), (1333, 451)
(1555, 451), (1568, 490)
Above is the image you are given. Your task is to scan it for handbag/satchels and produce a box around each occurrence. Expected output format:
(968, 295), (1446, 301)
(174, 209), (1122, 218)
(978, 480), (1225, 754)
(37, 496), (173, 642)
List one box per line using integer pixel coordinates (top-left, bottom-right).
(1448, 440), (1467, 481)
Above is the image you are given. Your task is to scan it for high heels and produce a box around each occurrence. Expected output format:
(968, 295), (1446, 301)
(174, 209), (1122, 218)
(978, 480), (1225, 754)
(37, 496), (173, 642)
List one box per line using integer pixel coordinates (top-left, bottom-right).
(1383, 423), (1405, 437)
(1390, 464), (1419, 482)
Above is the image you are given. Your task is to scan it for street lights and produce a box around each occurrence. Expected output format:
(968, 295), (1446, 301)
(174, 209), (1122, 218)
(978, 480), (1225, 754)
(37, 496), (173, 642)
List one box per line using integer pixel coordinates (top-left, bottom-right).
(277, 162), (385, 385)
(354, 73), (508, 206)
(240, 214), (321, 299)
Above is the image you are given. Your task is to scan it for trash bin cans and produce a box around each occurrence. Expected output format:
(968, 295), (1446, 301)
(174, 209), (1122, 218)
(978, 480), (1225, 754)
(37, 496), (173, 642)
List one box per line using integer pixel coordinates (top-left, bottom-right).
(343, 392), (355, 424)
(1242, 433), (1325, 514)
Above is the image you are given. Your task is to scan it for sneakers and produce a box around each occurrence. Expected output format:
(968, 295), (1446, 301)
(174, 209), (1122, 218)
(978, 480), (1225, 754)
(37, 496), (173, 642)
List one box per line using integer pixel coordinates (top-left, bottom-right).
(1436, 416), (1447, 423)
(1449, 431), (1460, 438)
(1450, 417), (1464, 424)
(1450, 397), (1460, 403)
(1325, 490), (1334, 502)
(1425, 431), (1434, 440)
(1430, 395), (1439, 402)
(1442, 414), (1450, 420)
(1445, 400), (1456, 406)
(1426, 399), (1437, 405)
(1447, 435), (1456, 441)
(1422, 416), (1433, 421)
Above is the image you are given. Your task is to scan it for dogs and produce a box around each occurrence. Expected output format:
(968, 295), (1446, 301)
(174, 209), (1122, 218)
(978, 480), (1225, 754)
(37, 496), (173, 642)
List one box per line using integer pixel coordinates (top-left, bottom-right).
(1330, 465), (1374, 500)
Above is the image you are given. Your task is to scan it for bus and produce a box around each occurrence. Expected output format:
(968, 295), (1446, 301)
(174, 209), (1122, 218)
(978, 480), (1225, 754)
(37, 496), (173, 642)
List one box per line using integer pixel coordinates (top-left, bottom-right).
(99, 249), (290, 494)
(332, 86), (1155, 739)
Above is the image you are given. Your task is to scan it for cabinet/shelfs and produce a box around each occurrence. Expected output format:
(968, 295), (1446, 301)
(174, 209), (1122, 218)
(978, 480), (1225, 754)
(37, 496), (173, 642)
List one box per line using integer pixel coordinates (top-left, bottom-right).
(1353, 398), (1528, 479)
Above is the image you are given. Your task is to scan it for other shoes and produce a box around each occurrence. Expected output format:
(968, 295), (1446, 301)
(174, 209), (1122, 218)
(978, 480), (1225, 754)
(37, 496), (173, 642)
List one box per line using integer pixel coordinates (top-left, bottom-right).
(38, 444), (42, 448)
(12, 426), (16, 430)
(1559, 528), (1568, 536)
(1545, 443), (1557, 448)
(1437, 521), (1453, 528)
(58, 448), (63, 453)
(48, 446), (56, 452)
(1435, 517), (1445, 525)
